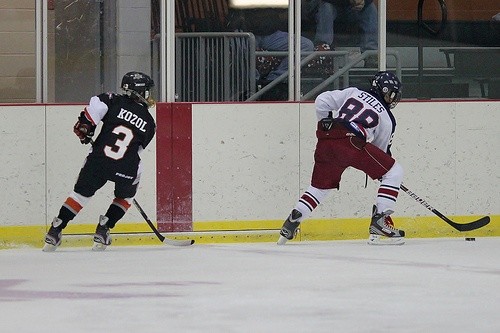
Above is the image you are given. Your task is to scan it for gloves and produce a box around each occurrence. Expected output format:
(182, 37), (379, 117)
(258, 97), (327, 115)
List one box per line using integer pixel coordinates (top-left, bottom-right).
(73, 111), (96, 145)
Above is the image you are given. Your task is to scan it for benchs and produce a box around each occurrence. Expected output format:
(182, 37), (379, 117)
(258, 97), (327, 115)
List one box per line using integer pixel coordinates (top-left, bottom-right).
(301, 19), (500, 46)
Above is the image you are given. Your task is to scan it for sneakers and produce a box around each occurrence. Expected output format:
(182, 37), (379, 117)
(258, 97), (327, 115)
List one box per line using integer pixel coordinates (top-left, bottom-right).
(41, 217), (67, 251)
(91, 214), (112, 250)
(277, 209), (302, 244)
(367, 204), (406, 245)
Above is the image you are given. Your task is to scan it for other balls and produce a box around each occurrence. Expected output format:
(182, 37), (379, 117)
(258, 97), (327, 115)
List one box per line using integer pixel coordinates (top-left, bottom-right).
(465, 238), (475, 241)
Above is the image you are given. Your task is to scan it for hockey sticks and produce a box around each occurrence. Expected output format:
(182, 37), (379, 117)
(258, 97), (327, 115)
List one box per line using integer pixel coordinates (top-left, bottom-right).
(399, 184), (491, 232)
(88, 135), (196, 247)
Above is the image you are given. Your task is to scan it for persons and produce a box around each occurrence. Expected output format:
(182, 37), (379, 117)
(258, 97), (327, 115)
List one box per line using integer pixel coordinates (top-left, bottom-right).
(277, 71), (406, 246)
(306, 0), (378, 52)
(216, 0), (315, 93)
(43, 71), (157, 251)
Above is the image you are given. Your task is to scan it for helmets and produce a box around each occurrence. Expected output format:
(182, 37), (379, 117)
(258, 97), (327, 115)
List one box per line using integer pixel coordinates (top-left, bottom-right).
(120, 72), (154, 97)
(370, 71), (403, 108)
